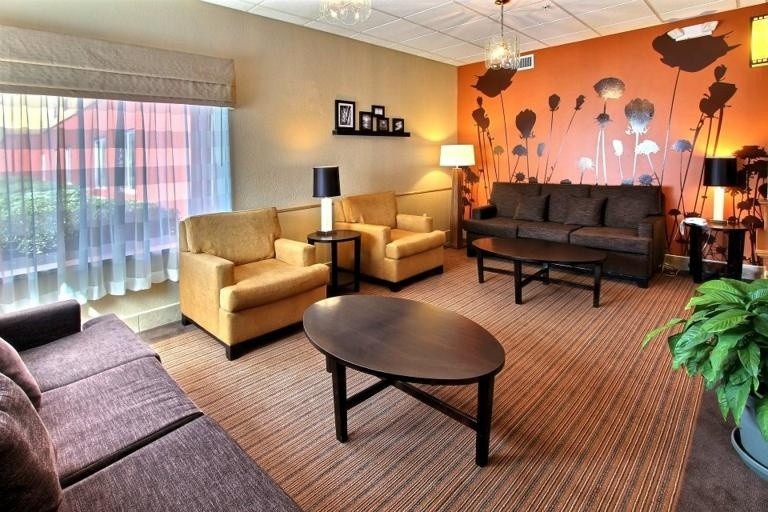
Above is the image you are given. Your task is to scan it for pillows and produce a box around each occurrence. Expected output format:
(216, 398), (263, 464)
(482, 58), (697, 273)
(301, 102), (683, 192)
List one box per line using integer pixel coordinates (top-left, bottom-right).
(560, 192), (609, 229)
(0, 373), (71, 512)
(508, 189), (552, 225)
(0, 334), (45, 404)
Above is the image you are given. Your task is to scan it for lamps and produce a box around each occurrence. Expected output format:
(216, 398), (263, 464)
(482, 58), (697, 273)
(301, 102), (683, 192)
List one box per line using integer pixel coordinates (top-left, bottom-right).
(745, 12), (768, 69)
(311, 164), (343, 236)
(481, 0), (521, 72)
(437, 143), (477, 250)
(701, 155), (738, 226)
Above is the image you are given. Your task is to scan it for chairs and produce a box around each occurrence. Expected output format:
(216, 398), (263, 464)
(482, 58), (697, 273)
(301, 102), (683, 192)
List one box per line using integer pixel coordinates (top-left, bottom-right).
(172, 205), (332, 362)
(328, 189), (448, 294)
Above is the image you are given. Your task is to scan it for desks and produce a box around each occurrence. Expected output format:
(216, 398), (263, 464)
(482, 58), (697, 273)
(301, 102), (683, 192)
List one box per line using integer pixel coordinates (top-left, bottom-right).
(305, 227), (365, 300)
(678, 213), (752, 286)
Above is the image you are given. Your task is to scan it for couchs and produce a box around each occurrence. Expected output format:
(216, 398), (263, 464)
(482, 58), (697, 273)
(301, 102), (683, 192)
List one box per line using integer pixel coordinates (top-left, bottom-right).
(461, 177), (670, 292)
(0, 286), (306, 511)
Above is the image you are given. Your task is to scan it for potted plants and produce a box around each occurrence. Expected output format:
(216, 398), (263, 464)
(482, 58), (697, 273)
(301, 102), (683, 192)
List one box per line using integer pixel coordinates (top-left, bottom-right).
(636, 272), (768, 485)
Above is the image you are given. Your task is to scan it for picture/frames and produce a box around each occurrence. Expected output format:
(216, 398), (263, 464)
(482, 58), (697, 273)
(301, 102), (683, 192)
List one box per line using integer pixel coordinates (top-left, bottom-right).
(331, 97), (407, 135)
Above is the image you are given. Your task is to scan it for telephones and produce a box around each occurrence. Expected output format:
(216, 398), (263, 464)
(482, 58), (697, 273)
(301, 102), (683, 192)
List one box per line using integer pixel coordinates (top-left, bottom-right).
(685, 217), (708, 226)
